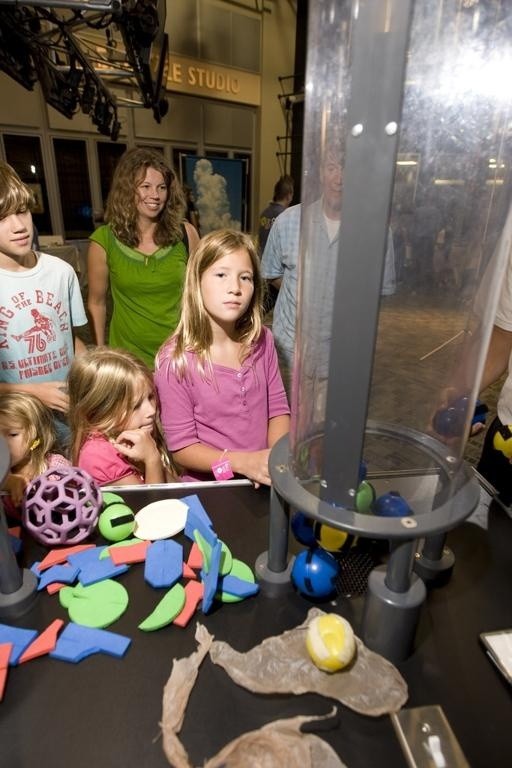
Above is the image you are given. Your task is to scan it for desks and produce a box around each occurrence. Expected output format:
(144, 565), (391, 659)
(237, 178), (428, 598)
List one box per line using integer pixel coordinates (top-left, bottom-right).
(0, 466), (511, 767)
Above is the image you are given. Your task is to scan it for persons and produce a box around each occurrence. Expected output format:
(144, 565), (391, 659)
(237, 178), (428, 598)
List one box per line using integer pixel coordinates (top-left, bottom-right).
(258, 175), (294, 315)
(88, 149), (200, 371)
(261, 147), (396, 419)
(151, 229), (290, 489)
(67, 346), (181, 488)
(0, 389), (73, 508)
(0, 159), (88, 459)
(429, 204), (512, 507)
